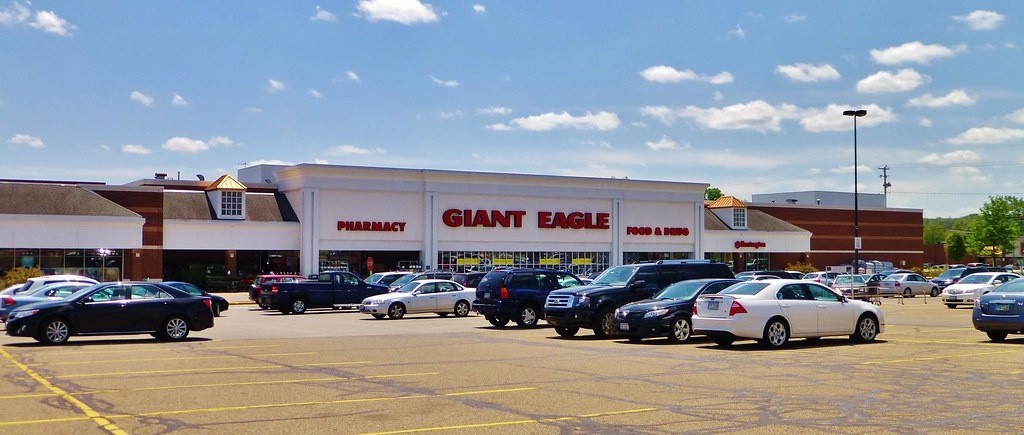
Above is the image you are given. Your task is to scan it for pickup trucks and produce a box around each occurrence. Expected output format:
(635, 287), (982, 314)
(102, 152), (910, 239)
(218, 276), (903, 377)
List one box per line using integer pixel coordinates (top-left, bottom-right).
(258, 271), (391, 315)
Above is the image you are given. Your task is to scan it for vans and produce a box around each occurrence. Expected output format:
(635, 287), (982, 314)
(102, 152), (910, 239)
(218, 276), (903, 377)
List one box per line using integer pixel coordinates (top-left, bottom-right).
(847, 259), (915, 278)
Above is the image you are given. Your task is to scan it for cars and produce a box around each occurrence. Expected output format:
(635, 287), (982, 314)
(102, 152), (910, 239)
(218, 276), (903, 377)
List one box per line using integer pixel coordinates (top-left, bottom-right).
(941, 272), (1024, 309)
(558, 271), (602, 287)
(734, 270), (887, 302)
(881, 269), (916, 279)
(144, 281), (230, 318)
(0, 273), (114, 299)
(358, 278), (477, 320)
(0, 281), (96, 326)
(691, 279), (885, 349)
(924, 262), (1014, 294)
(972, 277), (1024, 340)
(876, 273), (941, 298)
(612, 278), (746, 345)
(5, 281), (214, 346)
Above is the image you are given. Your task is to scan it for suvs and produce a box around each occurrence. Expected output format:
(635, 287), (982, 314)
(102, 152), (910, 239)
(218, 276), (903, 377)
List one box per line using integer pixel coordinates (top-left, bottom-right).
(543, 259), (737, 340)
(363, 269), (489, 289)
(472, 266), (587, 329)
(249, 275), (309, 311)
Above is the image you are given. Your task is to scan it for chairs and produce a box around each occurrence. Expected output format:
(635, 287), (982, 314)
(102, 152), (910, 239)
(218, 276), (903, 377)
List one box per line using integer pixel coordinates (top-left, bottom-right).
(783, 289), (796, 300)
(111, 289), (120, 300)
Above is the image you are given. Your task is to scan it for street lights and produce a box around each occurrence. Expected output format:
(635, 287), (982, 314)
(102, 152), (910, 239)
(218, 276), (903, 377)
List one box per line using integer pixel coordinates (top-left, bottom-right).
(843, 109), (867, 274)
